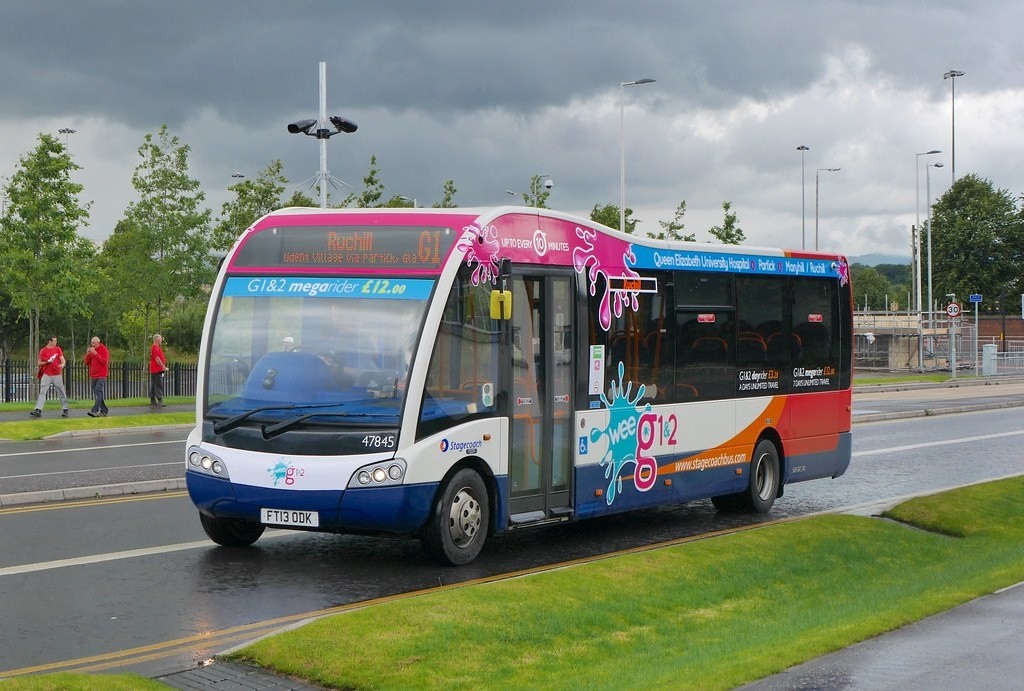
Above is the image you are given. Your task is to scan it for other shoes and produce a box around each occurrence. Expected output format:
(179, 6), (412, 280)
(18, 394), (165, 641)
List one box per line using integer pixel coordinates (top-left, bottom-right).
(151, 398), (166, 407)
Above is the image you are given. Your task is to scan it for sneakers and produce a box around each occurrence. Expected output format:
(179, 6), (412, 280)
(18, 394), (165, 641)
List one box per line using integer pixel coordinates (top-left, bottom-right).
(62, 410), (69, 417)
(30, 410), (41, 417)
(98, 412), (106, 417)
(87, 412), (97, 417)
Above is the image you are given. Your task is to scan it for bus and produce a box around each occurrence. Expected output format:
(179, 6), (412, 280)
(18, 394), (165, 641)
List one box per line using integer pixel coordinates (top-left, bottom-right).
(184, 206), (852, 567)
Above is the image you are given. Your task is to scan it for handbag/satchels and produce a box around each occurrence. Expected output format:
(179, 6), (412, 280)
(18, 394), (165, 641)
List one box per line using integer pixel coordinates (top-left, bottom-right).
(36, 368), (44, 380)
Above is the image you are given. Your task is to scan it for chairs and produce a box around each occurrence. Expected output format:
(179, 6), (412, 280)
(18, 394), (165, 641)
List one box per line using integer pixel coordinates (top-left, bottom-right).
(607, 317), (828, 403)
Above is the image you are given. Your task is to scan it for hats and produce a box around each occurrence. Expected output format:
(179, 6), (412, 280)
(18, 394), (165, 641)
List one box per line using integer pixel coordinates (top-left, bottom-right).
(282, 336), (294, 344)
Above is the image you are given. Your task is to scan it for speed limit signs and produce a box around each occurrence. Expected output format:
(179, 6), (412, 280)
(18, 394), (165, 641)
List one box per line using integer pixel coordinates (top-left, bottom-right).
(945, 302), (960, 317)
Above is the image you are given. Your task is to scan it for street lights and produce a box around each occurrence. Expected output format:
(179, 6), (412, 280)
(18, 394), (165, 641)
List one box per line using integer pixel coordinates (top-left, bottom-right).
(619, 78), (657, 233)
(58, 128), (77, 205)
(505, 174), (553, 208)
(287, 116), (358, 209)
(926, 162), (945, 329)
(796, 145), (809, 250)
(815, 168), (841, 252)
(917, 150), (943, 336)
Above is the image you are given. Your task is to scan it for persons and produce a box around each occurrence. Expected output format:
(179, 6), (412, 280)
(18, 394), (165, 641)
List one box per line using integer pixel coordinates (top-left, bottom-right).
(30, 334), (69, 418)
(84, 336), (109, 417)
(149, 333), (169, 407)
(281, 336), (296, 352)
